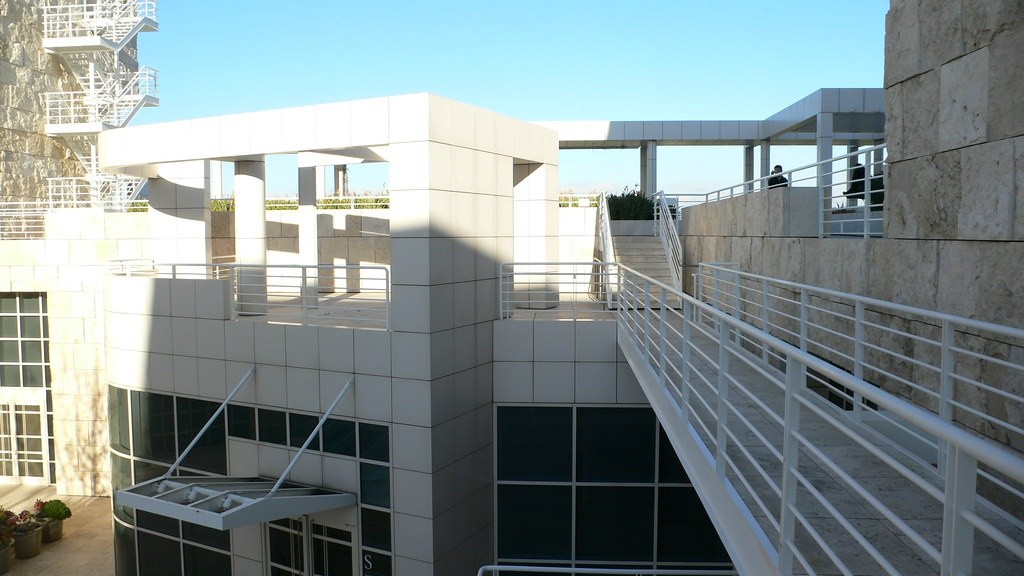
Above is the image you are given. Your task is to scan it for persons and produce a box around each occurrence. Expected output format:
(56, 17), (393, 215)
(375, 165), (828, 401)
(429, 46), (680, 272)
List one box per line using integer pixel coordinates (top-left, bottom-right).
(768, 165), (788, 190)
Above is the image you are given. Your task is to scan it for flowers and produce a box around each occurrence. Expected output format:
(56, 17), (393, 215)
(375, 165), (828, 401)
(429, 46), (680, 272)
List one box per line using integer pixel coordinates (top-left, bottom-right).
(15, 511), (36, 533)
(34, 499), (72, 521)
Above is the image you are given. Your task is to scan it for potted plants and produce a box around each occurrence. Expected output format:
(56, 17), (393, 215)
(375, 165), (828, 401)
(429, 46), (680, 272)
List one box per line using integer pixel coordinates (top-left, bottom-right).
(0, 526), (15, 576)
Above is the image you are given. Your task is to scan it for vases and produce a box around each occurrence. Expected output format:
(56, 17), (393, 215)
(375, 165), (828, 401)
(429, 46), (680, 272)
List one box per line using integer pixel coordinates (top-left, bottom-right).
(34, 517), (64, 542)
(13, 522), (42, 557)
